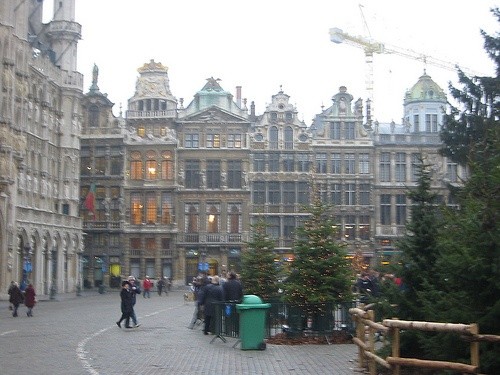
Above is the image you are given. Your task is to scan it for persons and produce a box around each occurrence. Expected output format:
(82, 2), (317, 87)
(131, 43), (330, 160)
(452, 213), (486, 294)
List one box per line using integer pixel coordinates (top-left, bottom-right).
(7, 280), (36, 317)
(117, 269), (407, 337)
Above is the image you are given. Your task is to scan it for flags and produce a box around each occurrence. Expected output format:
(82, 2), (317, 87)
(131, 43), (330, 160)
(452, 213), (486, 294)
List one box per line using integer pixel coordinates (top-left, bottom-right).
(84, 175), (96, 222)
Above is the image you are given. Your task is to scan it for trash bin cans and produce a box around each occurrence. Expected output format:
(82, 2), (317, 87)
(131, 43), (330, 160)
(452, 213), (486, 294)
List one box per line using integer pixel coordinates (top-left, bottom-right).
(236, 295), (271, 350)
